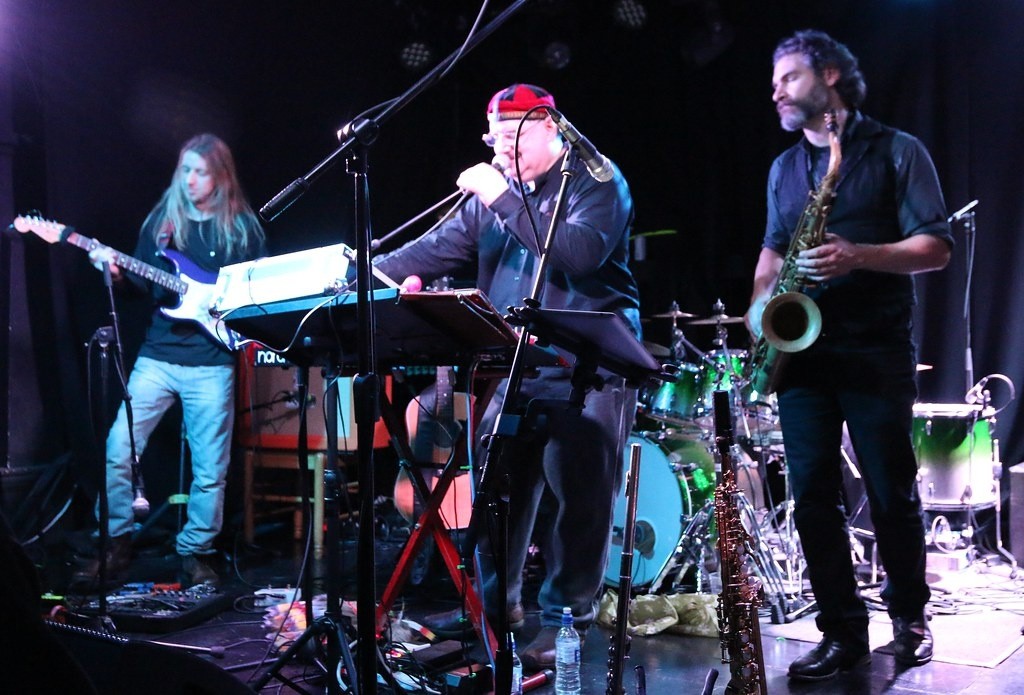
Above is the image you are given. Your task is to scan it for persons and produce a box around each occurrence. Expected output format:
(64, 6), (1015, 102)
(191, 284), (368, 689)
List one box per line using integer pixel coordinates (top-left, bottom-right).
(371, 84), (642, 668)
(751, 32), (955, 680)
(90, 134), (267, 557)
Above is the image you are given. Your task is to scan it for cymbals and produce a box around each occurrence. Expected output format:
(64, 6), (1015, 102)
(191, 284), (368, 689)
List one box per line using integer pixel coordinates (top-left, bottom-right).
(687, 315), (744, 325)
(916, 362), (935, 372)
(651, 311), (696, 318)
(642, 341), (671, 356)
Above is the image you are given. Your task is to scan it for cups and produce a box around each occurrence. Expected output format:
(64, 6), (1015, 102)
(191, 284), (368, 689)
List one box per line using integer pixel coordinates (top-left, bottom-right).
(709, 572), (723, 595)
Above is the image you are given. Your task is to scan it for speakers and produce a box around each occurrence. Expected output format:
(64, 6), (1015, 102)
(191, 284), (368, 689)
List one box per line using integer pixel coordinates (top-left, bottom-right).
(232, 342), (393, 452)
(45, 619), (260, 695)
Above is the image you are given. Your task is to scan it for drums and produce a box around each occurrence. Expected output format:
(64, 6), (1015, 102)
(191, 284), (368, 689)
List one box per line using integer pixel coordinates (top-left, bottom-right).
(602, 430), (765, 592)
(695, 349), (773, 431)
(750, 418), (850, 456)
(909, 402), (998, 512)
(636, 360), (702, 428)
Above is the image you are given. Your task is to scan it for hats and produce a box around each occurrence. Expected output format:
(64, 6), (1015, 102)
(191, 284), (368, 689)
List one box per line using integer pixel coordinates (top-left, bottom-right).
(487, 84), (554, 119)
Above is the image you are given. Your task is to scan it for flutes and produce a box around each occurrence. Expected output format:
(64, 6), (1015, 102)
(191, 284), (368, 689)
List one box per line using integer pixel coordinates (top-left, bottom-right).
(606, 443), (642, 695)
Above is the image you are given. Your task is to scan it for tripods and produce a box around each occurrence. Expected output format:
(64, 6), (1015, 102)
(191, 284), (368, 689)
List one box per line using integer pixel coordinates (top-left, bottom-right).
(650, 324), (807, 626)
(253, 338), (407, 695)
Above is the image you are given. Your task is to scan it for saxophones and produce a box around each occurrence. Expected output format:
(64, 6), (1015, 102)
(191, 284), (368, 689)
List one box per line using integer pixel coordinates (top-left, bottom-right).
(747, 108), (843, 396)
(711, 390), (767, 695)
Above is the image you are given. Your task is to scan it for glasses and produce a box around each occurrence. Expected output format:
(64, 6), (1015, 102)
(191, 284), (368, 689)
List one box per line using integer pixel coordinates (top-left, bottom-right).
(482, 121), (539, 147)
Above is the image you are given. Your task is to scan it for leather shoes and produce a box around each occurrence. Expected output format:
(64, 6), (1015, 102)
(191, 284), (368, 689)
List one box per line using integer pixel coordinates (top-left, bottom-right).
(179, 556), (221, 590)
(423, 600), (524, 637)
(519, 628), (586, 669)
(787, 637), (871, 681)
(892, 609), (933, 664)
(75, 537), (130, 582)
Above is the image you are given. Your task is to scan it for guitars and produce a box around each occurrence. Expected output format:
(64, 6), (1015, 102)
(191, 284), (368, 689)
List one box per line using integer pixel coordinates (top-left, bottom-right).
(393, 213), (476, 531)
(13, 213), (250, 353)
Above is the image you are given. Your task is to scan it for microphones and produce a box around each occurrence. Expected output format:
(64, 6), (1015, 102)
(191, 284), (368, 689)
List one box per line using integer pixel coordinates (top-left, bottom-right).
(465, 154), (511, 194)
(284, 391), (316, 406)
(547, 106), (614, 182)
(965, 377), (989, 405)
(618, 524), (645, 546)
(131, 475), (149, 516)
(947, 199), (980, 224)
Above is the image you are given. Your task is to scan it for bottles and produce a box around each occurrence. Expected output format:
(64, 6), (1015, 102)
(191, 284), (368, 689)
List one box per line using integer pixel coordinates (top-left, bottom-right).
(555, 606), (581, 695)
(506, 632), (523, 695)
(972, 554), (1001, 566)
